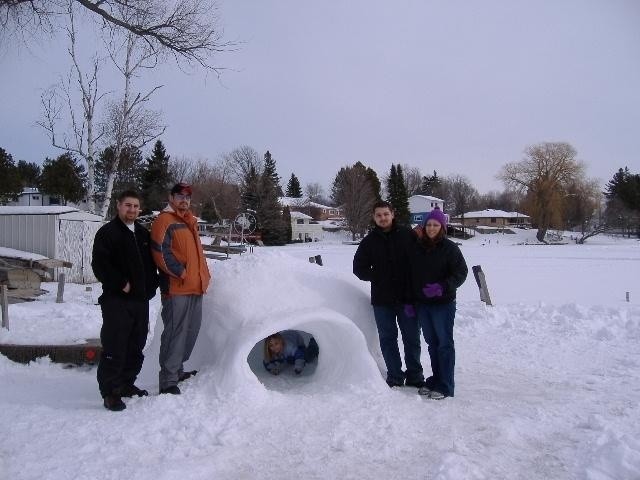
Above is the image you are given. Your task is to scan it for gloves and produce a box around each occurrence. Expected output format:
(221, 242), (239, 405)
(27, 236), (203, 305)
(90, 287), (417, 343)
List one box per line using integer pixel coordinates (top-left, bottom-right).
(403, 305), (415, 318)
(422, 283), (443, 298)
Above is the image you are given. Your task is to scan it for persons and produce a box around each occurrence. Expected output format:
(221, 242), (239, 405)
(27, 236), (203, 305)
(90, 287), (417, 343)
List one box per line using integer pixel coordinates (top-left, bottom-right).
(353, 201), (426, 389)
(150, 183), (212, 394)
(263, 331), (319, 376)
(414, 207), (468, 399)
(91, 191), (158, 411)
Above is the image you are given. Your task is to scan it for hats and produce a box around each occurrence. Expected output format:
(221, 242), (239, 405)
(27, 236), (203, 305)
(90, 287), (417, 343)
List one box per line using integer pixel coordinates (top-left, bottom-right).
(172, 182), (192, 193)
(425, 207), (447, 229)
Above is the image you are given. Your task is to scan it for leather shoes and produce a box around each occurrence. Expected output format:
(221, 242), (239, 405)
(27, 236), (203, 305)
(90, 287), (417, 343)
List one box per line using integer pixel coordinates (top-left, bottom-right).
(160, 370), (197, 394)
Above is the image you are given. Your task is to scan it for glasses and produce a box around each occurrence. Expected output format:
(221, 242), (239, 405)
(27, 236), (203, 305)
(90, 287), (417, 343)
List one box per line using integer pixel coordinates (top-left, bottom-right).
(175, 194), (193, 200)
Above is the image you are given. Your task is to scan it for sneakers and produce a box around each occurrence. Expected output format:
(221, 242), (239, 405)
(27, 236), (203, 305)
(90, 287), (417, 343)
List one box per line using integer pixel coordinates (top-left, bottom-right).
(386, 372), (451, 400)
(104, 384), (148, 411)
